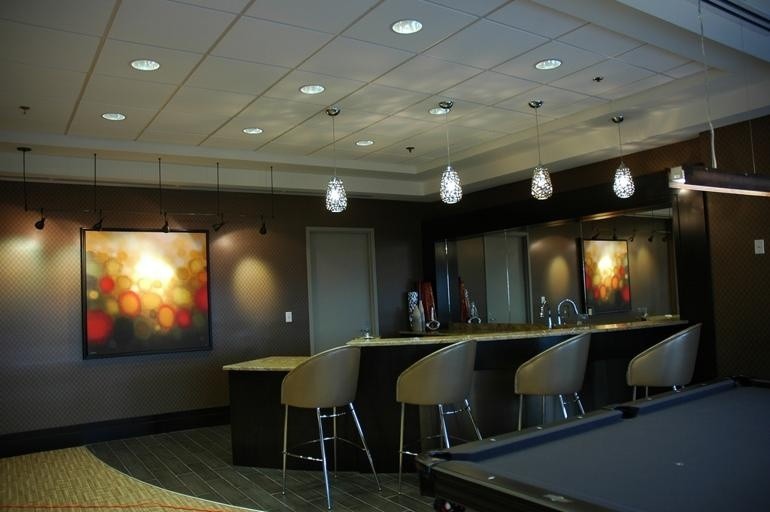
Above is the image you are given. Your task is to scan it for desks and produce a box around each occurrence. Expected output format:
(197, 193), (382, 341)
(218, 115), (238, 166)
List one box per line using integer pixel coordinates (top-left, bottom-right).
(412, 369), (770, 511)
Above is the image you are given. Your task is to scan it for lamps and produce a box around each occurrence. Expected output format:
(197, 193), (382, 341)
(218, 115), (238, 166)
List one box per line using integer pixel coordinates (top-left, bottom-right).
(586, 200), (673, 243)
(16, 146), (108, 230)
(156, 155), (276, 238)
(322, 105), (349, 216)
(438, 97), (464, 208)
(525, 98), (636, 201)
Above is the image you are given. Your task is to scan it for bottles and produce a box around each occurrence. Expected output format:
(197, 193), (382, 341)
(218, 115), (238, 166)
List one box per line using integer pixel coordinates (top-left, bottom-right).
(411, 301), (426, 331)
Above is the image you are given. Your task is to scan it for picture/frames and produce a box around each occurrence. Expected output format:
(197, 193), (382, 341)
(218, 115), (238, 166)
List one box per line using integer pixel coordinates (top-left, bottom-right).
(577, 235), (633, 316)
(80, 226), (215, 361)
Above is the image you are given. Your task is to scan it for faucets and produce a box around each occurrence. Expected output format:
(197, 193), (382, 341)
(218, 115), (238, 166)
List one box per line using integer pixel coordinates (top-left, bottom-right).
(538, 294), (554, 330)
(556, 298), (580, 326)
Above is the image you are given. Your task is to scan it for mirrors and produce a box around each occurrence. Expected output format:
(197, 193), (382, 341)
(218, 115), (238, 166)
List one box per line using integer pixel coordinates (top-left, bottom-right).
(434, 191), (681, 318)
